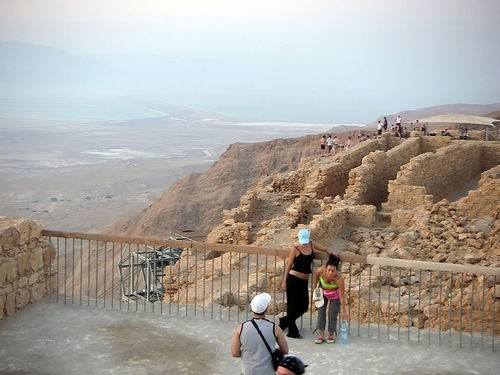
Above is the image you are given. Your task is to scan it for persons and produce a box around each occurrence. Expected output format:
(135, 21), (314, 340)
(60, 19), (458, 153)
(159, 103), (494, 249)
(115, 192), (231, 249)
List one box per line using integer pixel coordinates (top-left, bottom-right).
(273, 228), (350, 375)
(319, 114), (470, 158)
(231, 292), (289, 375)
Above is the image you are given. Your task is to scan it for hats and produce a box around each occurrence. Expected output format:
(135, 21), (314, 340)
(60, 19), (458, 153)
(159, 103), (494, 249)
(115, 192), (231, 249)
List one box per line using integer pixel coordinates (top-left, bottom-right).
(298, 230), (310, 245)
(250, 293), (271, 313)
(313, 287), (324, 308)
(278, 356), (308, 375)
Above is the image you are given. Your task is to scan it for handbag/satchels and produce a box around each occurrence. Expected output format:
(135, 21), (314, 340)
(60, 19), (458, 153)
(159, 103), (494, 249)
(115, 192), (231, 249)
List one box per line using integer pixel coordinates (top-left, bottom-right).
(271, 349), (284, 371)
(324, 289), (340, 300)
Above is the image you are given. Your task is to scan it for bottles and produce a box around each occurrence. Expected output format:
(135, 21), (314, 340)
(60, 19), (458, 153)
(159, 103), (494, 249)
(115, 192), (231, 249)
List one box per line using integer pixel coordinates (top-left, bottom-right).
(340, 321), (348, 344)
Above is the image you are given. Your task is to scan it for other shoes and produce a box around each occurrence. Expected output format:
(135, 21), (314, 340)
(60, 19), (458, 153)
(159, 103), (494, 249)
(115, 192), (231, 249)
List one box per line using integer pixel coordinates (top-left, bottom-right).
(327, 336), (336, 343)
(278, 318), (284, 330)
(315, 336), (325, 343)
(286, 334), (304, 339)
